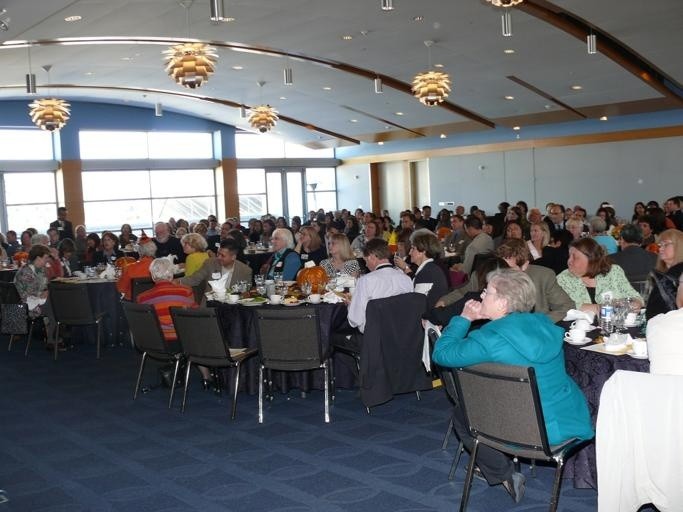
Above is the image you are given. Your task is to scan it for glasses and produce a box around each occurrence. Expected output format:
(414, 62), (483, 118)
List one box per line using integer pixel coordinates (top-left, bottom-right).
(655, 241), (674, 249)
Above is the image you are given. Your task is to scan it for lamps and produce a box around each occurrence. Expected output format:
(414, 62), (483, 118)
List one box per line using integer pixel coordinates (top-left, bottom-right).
(374, 74), (384, 94)
(26, 42), (37, 94)
(380, 0), (394, 11)
(410, 40), (453, 107)
(209, 0), (225, 23)
(27, 61), (70, 135)
(162, 0), (219, 92)
(578, 25), (602, 58)
(283, 50), (294, 85)
(244, 80), (279, 133)
(500, 8), (513, 37)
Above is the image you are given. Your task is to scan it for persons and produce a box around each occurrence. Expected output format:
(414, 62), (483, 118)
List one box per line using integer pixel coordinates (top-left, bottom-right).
(1, 200), (528, 391)
(431, 195), (683, 504)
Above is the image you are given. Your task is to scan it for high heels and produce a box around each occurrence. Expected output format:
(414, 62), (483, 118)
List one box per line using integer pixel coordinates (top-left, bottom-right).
(201, 376), (217, 391)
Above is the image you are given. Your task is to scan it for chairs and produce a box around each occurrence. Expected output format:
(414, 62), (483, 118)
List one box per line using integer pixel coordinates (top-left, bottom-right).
(251, 305), (335, 424)
(330, 292), (426, 416)
(595, 369), (682, 512)
(627, 275), (652, 304)
(453, 361), (596, 512)
(169, 304), (258, 421)
(121, 300), (186, 408)
(425, 324), (535, 481)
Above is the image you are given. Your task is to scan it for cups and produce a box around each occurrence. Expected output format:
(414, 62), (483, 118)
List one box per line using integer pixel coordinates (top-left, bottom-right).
(602, 334), (647, 359)
(269, 295), (283, 303)
(394, 253), (400, 268)
(571, 318), (592, 329)
(626, 312), (637, 323)
(308, 294), (322, 301)
(565, 329), (585, 342)
(231, 271), (283, 296)
(83, 258), (120, 280)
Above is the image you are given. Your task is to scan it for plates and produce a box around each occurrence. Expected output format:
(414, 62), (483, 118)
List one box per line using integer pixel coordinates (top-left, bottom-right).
(585, 326), (595, 333)
(306, 301), (322, 304)
(621, 322), (640, 328)
(239, 297), (267, 307)
(562, 337), (592, 345)
(281, 301), (305, 306)
(269, 302), (281, 306)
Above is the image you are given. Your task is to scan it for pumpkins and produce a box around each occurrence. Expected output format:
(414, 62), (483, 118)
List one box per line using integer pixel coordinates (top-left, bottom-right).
(115, 256), (135, 269)
(297, 266), (327, 293)
(14, 251), (28, 260)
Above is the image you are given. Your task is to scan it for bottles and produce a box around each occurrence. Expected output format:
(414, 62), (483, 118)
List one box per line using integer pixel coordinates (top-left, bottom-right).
(601, 295), (613, 333)
(613, 298), (632, 328)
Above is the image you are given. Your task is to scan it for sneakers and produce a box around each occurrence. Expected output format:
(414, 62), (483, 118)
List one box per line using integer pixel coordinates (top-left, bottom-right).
(506, 471), (526, 503)
(40, 336), (69, 353)
(463, 463), (489, 482)
